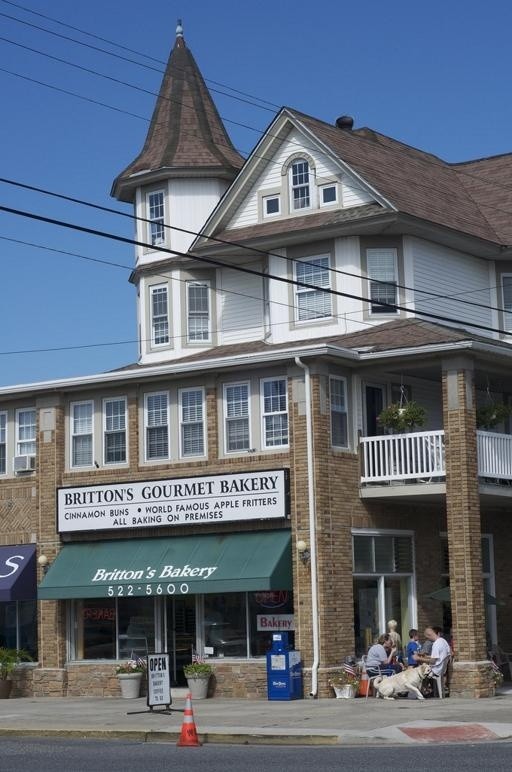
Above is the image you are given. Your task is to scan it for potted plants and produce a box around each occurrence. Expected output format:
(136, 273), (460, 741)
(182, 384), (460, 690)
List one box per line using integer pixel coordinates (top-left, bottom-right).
(0, 646), (34, 698)
(476, 404), (510, 431)
(184, 661), (216, 700)
(376, 399), (429, 433)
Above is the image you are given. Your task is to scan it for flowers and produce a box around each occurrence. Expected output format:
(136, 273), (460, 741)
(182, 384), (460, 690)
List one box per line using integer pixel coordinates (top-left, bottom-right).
(328, 670), (360, 688)
(113, 658), (144, 673)
(187, 653), (207, 669)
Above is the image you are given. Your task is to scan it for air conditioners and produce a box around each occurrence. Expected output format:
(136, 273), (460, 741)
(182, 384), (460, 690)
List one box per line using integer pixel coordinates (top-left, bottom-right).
(14, 455), (36, 472)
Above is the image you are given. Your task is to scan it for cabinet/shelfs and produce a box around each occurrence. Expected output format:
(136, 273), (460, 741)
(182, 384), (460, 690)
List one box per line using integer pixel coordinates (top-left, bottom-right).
(84, 624), (116, 657)
(120, 634), (194, 682)
(173, 607), (196, 634)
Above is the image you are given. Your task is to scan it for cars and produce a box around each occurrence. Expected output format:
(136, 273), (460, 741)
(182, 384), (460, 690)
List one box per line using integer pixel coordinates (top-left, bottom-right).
(204, 617), (245, 659)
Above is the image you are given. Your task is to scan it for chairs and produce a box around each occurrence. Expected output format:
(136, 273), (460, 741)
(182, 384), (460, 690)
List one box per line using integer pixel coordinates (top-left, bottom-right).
(421, 430), (446, 484)
(417, 654), (451, 699)
(362, 654), (395, 700)
(486, 644), (511, 680)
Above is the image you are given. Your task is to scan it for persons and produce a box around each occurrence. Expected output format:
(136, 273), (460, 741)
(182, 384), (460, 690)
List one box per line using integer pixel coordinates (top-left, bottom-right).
(365, 620), (452, 698)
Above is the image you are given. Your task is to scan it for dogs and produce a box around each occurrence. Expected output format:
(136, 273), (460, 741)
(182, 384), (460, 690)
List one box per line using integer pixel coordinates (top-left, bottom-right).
(373, 662), (435, 702)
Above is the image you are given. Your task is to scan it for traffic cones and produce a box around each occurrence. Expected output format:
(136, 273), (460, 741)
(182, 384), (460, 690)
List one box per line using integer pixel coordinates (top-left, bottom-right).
(176, 693), (203, 748)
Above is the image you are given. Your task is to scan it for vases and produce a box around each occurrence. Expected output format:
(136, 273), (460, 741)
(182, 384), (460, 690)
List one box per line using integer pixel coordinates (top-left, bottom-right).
(333, 684), (357, 699)
(117, 673), (142, 699)
